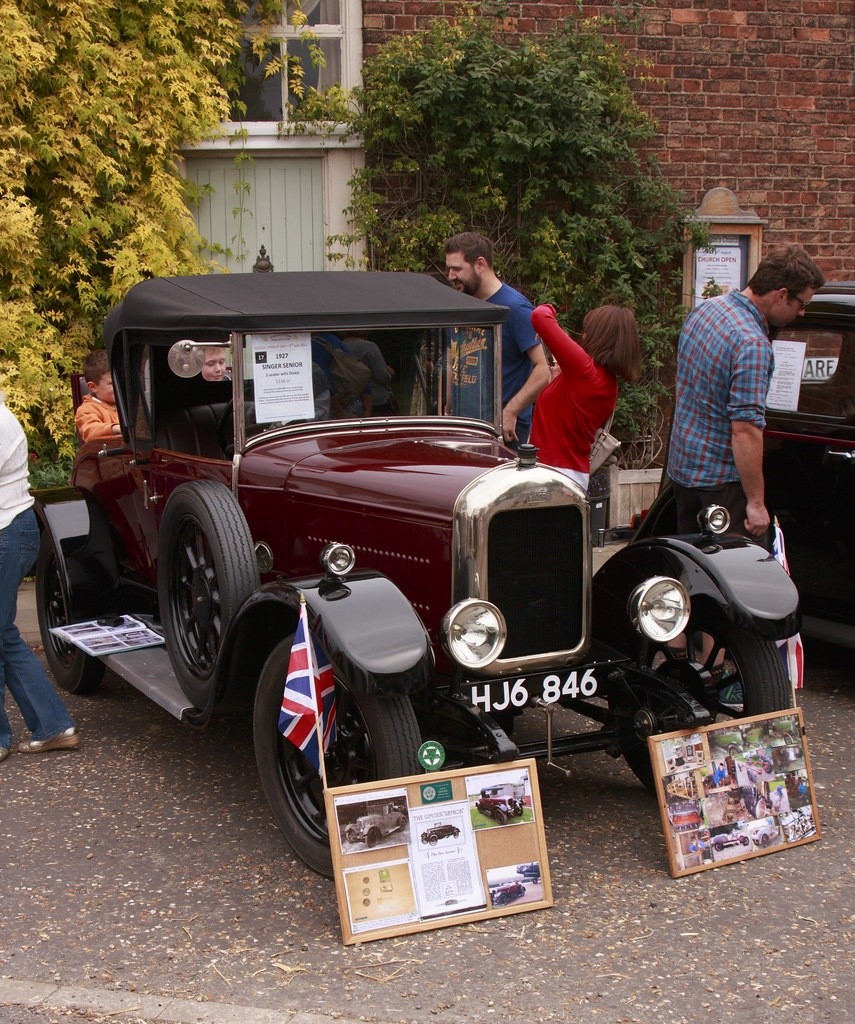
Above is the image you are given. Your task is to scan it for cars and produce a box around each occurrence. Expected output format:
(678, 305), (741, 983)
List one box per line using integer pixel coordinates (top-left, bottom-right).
(710, 731), (744, 758)
(490, 880), (527, 905)
(713, 834), (749, 852)
(475, 786), (524, 825)
(769, 720), (799, 745)
(628, 281), (855, 651)
(345, 802), (408, 848)
(752, 826), (781, 847)
(420, 822), (460, 846)
(28, 268), (804, 881)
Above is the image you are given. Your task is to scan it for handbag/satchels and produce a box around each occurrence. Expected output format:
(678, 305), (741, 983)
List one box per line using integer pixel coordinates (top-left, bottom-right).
(588, 405), (621, 476)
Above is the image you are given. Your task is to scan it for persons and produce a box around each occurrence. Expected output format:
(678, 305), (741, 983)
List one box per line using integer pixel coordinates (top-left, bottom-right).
(755, 796), (771, 819)
(266, 332), (399, 430)
(529, 302), (642, 492)
(200, 348), (231, 381)
(666, 247), (826, 717)
(0, 402), (81, 762)
(713, 762), (725, 788)
(73, 349), (122, 445)
(786, 774), (804, 796)
(431, 231), (552, 453)
(688, 837), (706, 853)
(772, 746), (787, 774)
(763, 720), (776, 735)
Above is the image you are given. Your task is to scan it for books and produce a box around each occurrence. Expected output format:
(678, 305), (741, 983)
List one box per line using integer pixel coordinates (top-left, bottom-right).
(48, 614), (165, 658)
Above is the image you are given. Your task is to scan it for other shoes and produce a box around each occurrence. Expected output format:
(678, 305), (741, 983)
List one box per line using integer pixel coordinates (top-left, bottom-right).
(18, 730), (79, 754)
(0, 747), (10, 762)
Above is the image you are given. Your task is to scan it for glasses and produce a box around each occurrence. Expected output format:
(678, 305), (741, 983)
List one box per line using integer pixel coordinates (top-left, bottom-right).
(774, 288), (811, 312)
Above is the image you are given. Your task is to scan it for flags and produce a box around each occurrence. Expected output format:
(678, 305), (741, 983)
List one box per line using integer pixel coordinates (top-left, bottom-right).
(772, 529), (804, 690)
(276, 610), (338, 781)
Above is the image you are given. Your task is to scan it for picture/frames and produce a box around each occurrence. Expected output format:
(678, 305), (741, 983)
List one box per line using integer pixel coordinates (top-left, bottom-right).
(323, 758), (553, 946)
(647, 707), (822, 878)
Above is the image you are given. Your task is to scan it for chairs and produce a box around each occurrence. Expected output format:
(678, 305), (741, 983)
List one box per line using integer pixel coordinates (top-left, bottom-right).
(70, 374), (90, 447)
(166, 401), (255, 460)
